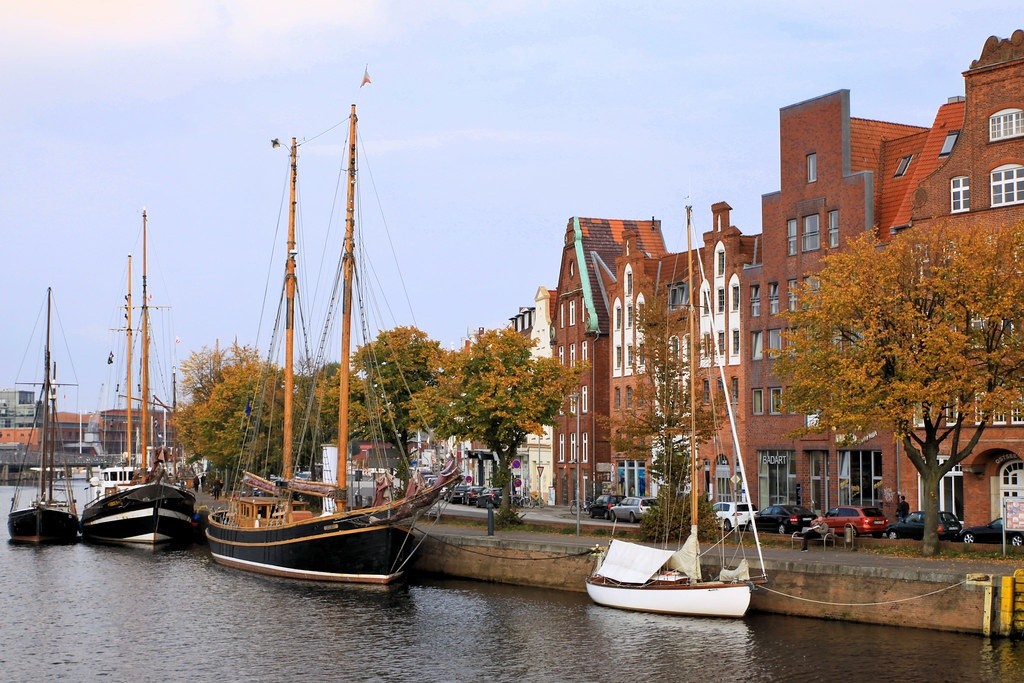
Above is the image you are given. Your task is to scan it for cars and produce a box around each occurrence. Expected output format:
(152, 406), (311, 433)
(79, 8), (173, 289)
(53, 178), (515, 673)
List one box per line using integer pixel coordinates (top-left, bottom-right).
(885, 511), (962, 541)
(296, 471), (312, 480)
(587, 494), (627, 520)
(957, 518), (1024, 547)
(811, 505), (888, 538)
(410, 467), (524, 509)
(609, 497), (659, 523)
(746, 504), (819, 534)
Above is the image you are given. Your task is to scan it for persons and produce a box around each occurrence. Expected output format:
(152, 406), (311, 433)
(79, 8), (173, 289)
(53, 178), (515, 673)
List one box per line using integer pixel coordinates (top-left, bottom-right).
(801, 516), (827, 553)
(190, 472), (205, 494)
(895, 493), (911, 521)
(210, 476), (224, 498)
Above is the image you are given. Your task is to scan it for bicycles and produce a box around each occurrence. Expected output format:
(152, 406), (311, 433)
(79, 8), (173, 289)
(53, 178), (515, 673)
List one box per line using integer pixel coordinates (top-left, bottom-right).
(513, 494), (531, 508)
(570, 496), (593, 515)
(528, 495), (545, 509)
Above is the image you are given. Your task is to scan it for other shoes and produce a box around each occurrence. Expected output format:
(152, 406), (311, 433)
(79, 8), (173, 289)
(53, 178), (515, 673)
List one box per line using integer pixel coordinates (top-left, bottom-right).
(214, 497), (219, 500)
(800, 548), (808, 552)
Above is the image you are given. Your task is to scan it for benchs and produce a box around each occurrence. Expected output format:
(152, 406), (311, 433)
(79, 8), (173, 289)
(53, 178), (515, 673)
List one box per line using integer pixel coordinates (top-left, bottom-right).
(791, 527), (835, 552)
(423, 505), (441, 522)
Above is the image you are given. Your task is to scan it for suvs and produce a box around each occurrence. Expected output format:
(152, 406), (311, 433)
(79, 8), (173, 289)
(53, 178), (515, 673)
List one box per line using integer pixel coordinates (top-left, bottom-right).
(712, 502), (758, 531)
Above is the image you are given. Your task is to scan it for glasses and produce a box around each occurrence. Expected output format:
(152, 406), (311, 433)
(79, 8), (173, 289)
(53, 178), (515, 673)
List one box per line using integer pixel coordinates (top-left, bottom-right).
(818, 521), (822, 523)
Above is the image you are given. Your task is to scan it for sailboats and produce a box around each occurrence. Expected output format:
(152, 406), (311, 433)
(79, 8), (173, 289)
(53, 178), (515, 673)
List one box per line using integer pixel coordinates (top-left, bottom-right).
(584, 205), (770, 617)
(88, 254), (167, 506)
(204, 104), (462, 595)
(8, 286), (80, 546)
(80, 210), (196, 546)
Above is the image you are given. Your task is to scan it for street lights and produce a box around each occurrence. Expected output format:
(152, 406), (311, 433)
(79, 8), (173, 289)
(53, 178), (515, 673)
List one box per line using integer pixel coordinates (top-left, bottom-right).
(568, 394), (580, 536)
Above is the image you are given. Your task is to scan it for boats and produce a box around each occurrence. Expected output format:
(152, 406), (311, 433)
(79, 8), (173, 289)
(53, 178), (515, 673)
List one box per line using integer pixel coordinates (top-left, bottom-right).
(168, 464), (195, 489)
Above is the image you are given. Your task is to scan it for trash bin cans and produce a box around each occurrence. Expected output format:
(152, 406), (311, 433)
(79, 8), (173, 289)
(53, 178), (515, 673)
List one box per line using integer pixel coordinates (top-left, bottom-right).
(843, 522), (856, 551)
